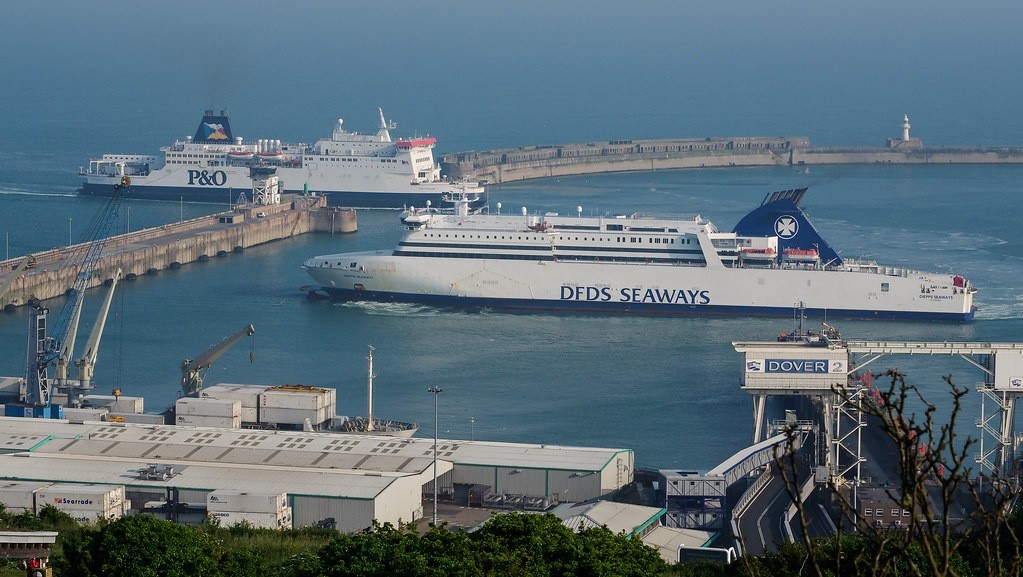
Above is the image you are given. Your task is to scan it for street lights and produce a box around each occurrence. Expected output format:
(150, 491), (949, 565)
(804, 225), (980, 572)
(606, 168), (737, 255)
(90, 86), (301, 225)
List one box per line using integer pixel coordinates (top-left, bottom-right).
(428, 385), (442, 527)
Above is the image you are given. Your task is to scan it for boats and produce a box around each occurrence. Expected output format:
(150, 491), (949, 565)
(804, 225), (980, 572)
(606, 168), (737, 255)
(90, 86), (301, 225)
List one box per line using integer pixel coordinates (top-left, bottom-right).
(75, 105), (488, 209)
(301, 183), (978, 323)
(0, 173), (419, 437)
